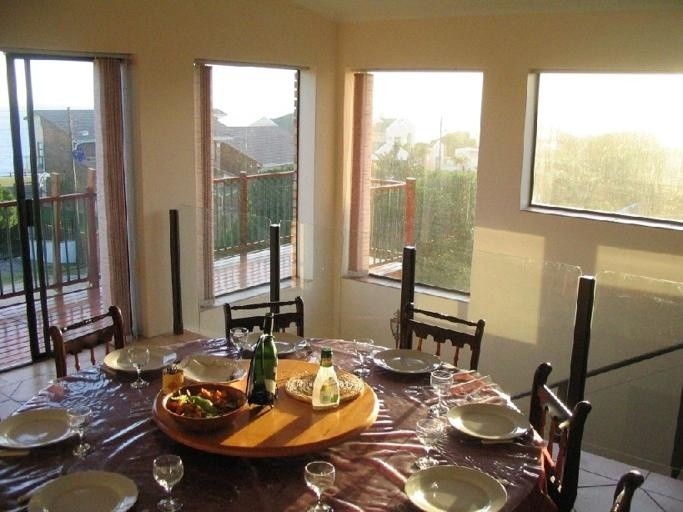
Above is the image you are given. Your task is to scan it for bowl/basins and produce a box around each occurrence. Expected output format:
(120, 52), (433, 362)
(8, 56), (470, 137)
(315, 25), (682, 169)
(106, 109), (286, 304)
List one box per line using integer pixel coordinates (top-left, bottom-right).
(160, 381), (249, 435)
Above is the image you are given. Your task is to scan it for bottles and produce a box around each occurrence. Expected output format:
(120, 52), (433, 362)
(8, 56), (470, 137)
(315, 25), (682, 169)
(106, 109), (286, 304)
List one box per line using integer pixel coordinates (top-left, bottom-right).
(243, 311), (280, 407)
(309, 344), (342, 412)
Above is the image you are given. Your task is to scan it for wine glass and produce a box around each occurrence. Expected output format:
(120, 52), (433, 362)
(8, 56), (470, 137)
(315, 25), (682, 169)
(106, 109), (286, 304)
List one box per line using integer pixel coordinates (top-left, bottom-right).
(64, 406), (94, 457)
(426, 369), (452, 418)
(303, 461), (337, 512)
(227, 326), (249, 360)
(414, 418), (446, 470)
(350, 336), (375, 376)
(127, 343), (153, 390)
(151, 453), (186, 512)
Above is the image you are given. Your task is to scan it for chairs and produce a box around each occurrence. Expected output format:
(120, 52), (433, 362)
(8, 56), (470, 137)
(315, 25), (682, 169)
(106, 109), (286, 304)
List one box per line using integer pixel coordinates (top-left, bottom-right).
(220, 296), (305, 340)
(610, 469), (644, 512)
(529, 360), (591, 511)
(47, 302), (126, 378)
(399, 300), (486, 373)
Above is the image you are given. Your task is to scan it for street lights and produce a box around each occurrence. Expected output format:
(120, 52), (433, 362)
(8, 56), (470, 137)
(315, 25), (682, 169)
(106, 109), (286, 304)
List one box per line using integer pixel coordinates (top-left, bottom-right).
(69, 129), (89, 232)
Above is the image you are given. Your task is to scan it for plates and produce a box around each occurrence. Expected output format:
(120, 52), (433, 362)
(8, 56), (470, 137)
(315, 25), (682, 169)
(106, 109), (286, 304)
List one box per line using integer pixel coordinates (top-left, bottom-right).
(178, 353), (246, 385)
(102, 345), (178, 373)
(403, 463), (508, 512)
(0, 406), (82, 448)
(372, 348), (444, 375)
(444, 401), (531, 442)
(29, 470), (140, 512)
(238, 327), (309, 356)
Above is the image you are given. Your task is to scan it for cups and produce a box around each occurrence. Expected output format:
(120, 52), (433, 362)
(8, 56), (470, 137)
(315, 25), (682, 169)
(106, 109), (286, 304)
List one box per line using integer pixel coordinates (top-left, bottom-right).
(161, 367), (185, 395)
